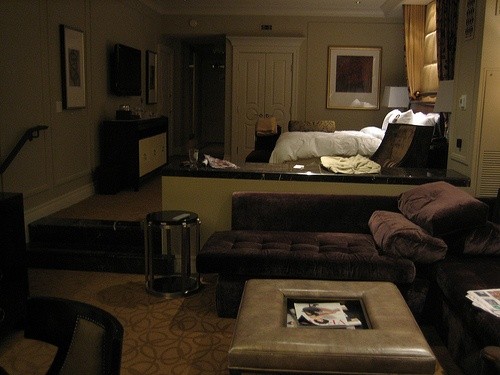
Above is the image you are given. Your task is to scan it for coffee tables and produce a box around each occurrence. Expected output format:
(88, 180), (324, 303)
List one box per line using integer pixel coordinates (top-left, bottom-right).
(227, 278), (445, 375)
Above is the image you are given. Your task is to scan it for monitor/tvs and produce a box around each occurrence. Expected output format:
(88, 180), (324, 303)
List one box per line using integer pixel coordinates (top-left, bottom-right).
(112, 43), (142, 96)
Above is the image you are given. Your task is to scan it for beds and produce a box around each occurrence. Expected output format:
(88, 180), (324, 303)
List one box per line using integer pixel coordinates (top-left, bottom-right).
(269, 106), (446, 164)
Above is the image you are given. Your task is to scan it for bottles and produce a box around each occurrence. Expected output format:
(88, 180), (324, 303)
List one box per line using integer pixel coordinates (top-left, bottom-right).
(134, 107), (141, 118)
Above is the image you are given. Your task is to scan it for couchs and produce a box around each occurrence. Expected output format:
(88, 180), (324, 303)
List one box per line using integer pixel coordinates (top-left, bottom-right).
(422, 190), (500, 375)
(196, 192), (491, 319)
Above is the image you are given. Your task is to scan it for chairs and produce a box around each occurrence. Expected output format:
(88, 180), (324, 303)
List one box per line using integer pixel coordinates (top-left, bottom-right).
(0, 296), (124, 375)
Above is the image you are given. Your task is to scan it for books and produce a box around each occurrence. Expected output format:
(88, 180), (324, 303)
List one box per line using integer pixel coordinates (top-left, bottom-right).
(290, 303), (361, 329)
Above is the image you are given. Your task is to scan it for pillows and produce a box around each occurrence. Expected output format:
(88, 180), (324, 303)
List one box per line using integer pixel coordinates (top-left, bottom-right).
(398, 181), (489, 242)
(368, 210), (448, 265)
(289, 120), (336, 132)
(382, 107), (440, 134)
(443, 221), (500, 254)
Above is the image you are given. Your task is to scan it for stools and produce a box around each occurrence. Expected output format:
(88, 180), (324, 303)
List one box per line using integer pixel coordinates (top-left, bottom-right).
(143, 210), (201, 298)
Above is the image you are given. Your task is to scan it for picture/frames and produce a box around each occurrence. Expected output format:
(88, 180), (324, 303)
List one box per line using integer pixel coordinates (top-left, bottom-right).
(144, 49), (158, 106)
(495, 0), (500, 16)
(325, 45), (382, 110)
(60, 24), (88, 111)
(463, 0), (476, 41)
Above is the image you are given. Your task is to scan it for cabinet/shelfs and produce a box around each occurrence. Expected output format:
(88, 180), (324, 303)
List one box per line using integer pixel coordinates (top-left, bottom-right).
(226, 35), (307, 160)
(99, 116), (169, 193)
(0, 191), (29, 340)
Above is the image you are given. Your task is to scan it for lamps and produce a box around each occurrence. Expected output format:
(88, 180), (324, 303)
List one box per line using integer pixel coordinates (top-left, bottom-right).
(382, 86), (410, 111)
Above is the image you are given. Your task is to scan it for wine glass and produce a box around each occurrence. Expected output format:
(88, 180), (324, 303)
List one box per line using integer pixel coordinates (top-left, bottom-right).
(188, 149), (199, 169)
